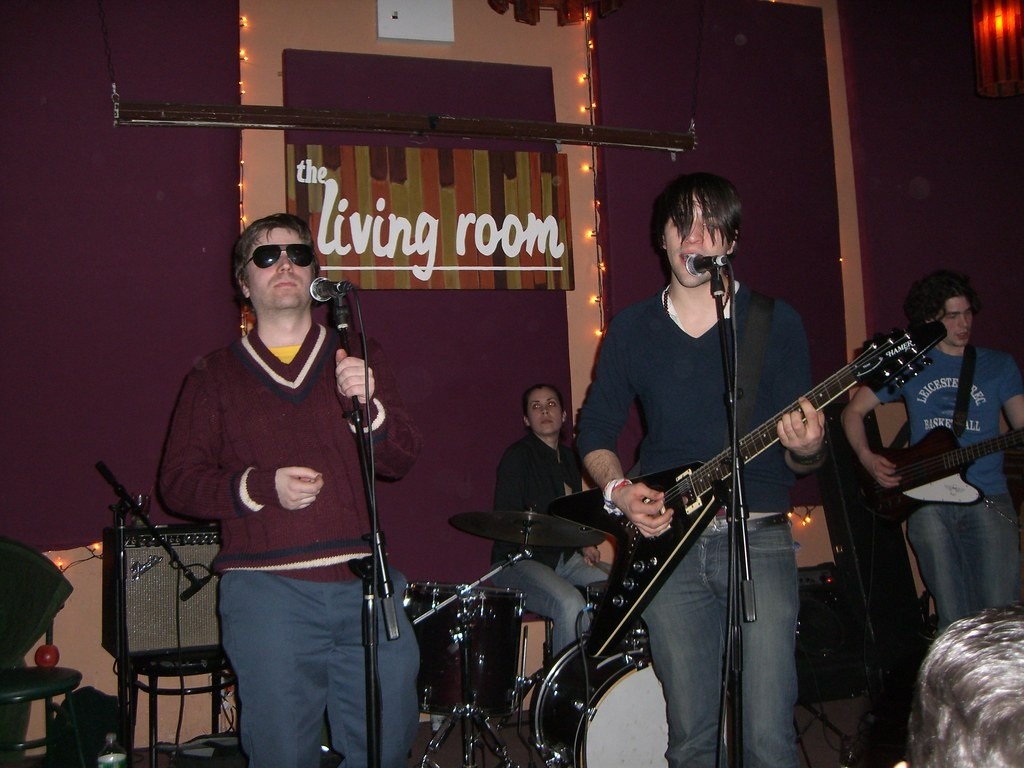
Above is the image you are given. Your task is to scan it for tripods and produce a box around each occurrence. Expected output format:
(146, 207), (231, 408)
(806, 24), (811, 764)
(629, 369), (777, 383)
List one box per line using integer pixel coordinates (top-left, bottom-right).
(410, 521), (533, 768)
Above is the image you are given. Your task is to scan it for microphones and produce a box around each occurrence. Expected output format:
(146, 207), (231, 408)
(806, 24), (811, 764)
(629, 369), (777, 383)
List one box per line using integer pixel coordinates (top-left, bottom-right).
(310, 277), (354, 302)
(180, 574), (213, 601)
(686, 252), (729, 277)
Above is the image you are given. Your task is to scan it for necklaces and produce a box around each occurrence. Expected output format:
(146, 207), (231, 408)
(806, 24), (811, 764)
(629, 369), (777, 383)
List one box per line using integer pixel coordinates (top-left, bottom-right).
(663, 284), (731, 317)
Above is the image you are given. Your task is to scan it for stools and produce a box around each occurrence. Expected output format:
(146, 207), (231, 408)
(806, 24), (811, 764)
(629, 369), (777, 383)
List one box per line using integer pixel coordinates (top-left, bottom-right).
(524, 584), (588, 669)
(0, 665), (86, 768)
(127, 650), (240, 766)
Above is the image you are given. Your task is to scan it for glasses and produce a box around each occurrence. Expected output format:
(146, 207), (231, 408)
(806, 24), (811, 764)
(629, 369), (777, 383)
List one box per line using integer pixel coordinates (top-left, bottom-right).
(243, 244), (314, 267)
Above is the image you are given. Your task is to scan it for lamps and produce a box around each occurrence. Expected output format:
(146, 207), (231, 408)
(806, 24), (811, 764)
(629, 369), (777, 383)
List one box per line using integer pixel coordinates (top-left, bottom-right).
(971, 0), (1024, 99)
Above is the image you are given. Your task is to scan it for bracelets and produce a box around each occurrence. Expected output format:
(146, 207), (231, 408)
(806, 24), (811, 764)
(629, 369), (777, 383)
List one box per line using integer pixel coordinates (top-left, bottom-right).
(602, 477), (632, 517)
(790, 440), (826, 465)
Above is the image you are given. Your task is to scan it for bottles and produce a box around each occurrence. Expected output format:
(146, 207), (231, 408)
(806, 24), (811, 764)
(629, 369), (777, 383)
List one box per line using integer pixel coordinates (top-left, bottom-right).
(97, 733), (126, 768)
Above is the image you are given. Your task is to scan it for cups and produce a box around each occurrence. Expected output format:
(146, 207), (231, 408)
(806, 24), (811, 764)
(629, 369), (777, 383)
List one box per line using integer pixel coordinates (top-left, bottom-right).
(430, 714), (448, 732)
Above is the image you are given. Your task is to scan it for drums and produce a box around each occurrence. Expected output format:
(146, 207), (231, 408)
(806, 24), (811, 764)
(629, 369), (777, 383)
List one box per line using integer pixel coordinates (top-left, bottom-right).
(400, 581), (527, 716)
(584, 579), (650, 643)
(528, 631), (670, 768)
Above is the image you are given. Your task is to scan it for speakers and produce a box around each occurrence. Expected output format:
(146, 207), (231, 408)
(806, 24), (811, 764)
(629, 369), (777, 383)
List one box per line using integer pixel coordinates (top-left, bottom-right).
(100, 522), (223, 664)
(794, 563), (871, 704)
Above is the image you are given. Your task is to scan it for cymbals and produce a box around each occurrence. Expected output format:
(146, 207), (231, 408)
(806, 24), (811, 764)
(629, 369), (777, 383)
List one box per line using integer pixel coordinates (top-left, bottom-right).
(448, 511), (605, 548)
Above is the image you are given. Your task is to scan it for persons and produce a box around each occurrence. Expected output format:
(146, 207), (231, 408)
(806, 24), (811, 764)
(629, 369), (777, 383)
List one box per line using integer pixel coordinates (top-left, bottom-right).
(892, 601), (1024, 768)
(841, 271), (1024, 632)
(575, 173), (830, 768)
(158, 213), (422, 767)
(488, 385), (611, 664)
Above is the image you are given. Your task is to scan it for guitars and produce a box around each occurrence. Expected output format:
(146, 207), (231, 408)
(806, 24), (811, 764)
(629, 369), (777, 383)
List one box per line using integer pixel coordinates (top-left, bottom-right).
(843, 426), (1024, 520)
(546, 318), (947, 658)
